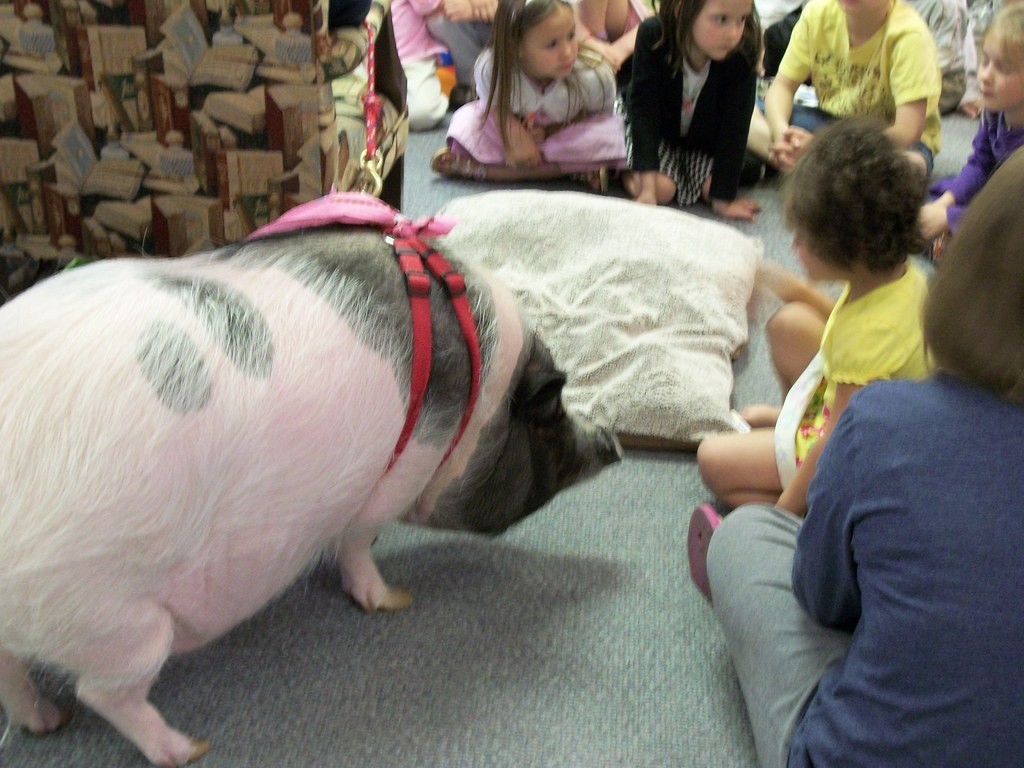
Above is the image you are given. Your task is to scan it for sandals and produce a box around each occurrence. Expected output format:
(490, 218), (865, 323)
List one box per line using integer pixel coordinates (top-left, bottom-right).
(572, 165), (607, 195)
(429, 148), (489, 185)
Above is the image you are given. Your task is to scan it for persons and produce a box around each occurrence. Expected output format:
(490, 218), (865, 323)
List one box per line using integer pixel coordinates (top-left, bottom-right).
(390, 1), (945, 224)
(689, 0), (1024, 768)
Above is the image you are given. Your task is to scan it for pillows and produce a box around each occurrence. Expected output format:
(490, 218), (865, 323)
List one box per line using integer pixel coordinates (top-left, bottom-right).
(445, 101), (626, 165)
(431, 188), (763, 446)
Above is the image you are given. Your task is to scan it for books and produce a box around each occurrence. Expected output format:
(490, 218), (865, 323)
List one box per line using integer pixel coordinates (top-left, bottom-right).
(0, 0), (412, 269)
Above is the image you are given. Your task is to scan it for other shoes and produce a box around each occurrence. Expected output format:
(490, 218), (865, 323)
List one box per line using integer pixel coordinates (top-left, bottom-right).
(688, 503), (725, 605)
(448, 82), (482, 111)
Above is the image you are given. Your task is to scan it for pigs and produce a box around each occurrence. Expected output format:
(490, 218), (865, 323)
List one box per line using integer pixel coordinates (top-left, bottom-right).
(1, 225), (625, 768)
